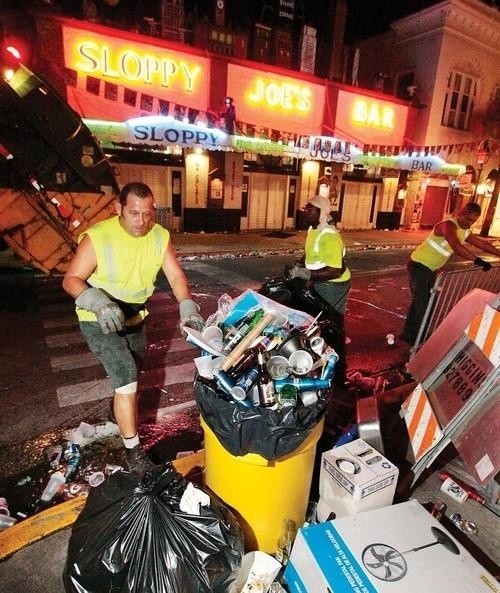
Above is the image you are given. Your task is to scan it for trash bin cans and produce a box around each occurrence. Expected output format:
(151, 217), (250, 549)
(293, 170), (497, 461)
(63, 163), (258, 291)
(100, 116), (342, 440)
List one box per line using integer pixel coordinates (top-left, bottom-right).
(193, 342), (335, 556)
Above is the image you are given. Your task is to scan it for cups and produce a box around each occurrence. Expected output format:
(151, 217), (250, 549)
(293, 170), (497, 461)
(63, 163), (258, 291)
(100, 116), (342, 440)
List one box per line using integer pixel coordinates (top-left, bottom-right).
(440, 476), (468, 503)
(386, 333), (395, 346)
(41, 474), (66, 501)
(89, 471), (106, 487)
(184, 309), (337, 409)
(0, 497), (17, 530)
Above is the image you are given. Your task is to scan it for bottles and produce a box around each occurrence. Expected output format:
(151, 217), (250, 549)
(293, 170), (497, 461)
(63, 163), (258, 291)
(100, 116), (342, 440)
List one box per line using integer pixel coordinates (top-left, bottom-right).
(437, 471), (486, 505)
(49, 444), (81, 483)
(276, 519), (298, 572)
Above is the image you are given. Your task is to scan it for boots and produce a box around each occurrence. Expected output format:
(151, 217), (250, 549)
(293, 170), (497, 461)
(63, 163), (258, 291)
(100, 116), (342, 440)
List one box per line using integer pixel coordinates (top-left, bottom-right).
(122, 442), (160, 478)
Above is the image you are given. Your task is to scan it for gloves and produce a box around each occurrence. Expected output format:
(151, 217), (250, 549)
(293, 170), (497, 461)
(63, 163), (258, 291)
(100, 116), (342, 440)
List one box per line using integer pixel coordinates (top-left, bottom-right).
(474, 257), (492, 272)
(73, 286), (126, 336)
(288, 265), (313, 282)
(179, 298), (206, 338)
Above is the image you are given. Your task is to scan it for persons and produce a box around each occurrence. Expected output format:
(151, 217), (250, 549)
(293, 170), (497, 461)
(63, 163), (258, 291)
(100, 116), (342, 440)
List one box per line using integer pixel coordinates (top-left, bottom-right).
(329, 175), (339, 206)
(398, 201), (500, 346)
(288, 195), (354, 375)
(302, 136), (342, 153)
(217, 96), (236, 135)
(62, 181), (206, 479)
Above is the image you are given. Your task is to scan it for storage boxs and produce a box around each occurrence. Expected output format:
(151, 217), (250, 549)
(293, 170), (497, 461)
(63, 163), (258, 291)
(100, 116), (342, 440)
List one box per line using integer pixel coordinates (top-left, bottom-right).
(282, 495), (500, 593)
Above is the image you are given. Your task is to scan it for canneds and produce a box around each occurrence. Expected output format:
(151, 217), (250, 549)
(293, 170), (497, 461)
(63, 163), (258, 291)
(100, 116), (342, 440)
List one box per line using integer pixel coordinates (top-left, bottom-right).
(49, 443), (81, 484)
(232, 366), (260, 401)
(104, 464), (124, 476)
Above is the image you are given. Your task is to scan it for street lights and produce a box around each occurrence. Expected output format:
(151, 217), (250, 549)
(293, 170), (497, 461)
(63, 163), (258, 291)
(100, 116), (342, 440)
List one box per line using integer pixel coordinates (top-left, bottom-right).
(471, 136), (491, 207)
